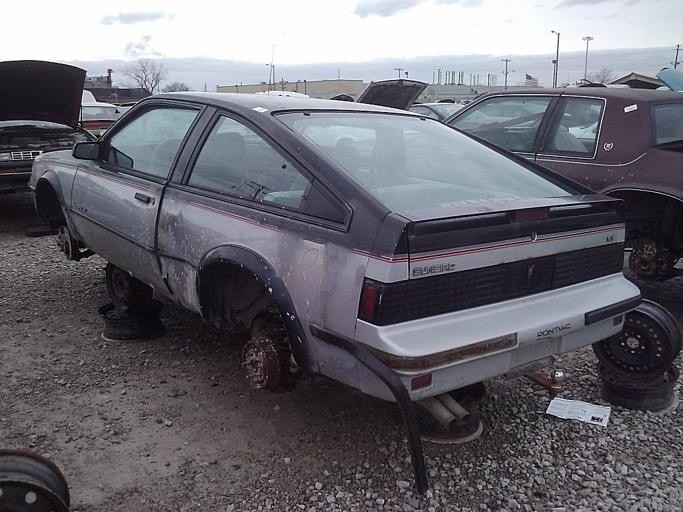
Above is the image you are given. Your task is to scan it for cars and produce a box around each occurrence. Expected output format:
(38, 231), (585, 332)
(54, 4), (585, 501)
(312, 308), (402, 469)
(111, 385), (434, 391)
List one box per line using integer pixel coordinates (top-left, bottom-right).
(74, 78), (683, 141)
(0, 60), (100, 198)
(27, 88), (643, 435)
(437, 84), (683, 283)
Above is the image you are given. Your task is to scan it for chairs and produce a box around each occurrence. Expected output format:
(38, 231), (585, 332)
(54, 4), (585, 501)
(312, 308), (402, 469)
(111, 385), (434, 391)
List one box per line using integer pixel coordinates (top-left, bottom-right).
(151, 132), (378, 205)
(542, 123), (590, 152)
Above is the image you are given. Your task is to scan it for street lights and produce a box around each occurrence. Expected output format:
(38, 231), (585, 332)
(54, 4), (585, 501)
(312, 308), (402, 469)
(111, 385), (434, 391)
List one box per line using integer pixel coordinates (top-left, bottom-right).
(551, 30), (561, 88)
(581, 36), (593, 79)
